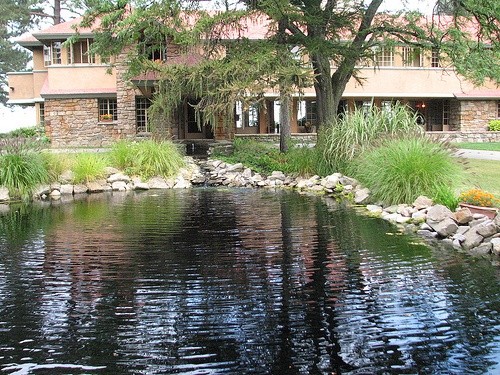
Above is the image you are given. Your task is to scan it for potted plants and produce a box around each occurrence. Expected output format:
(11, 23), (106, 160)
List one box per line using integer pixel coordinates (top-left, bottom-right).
(101, 113), (113, 122)
